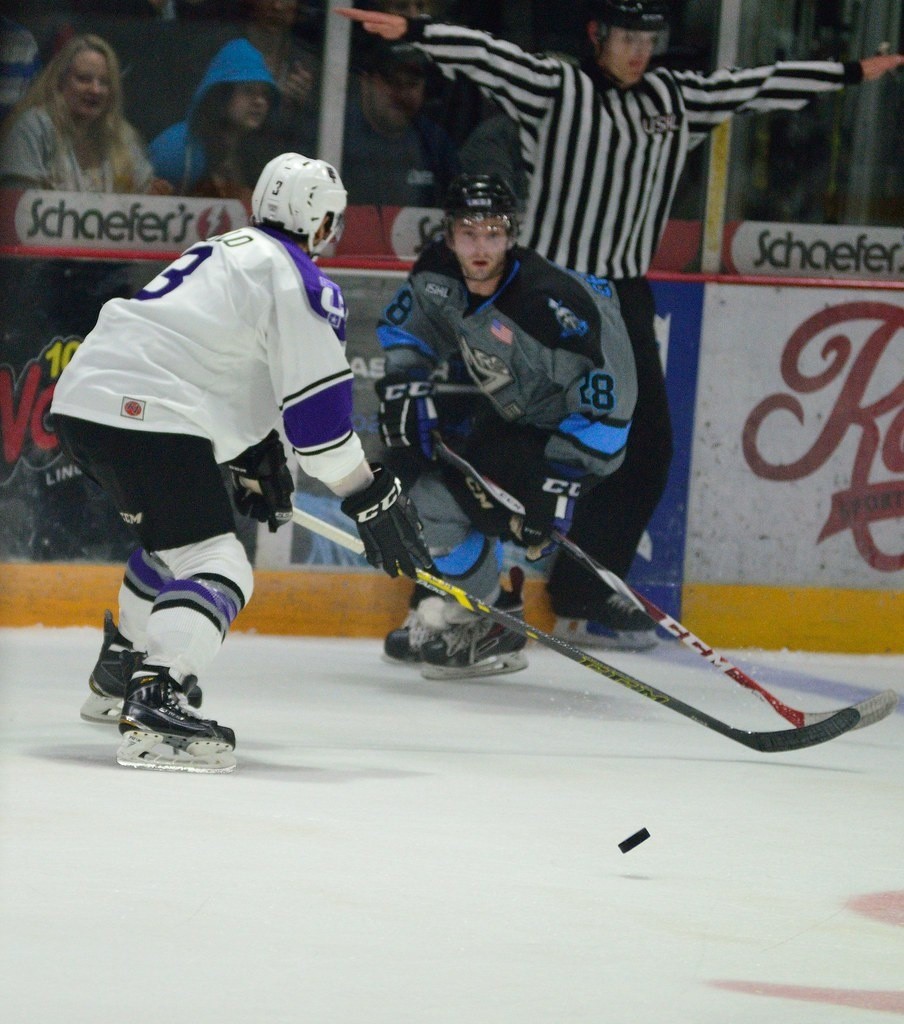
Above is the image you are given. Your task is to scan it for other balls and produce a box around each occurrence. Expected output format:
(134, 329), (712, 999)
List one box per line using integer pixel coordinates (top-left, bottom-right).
(617, 826), (652, 855)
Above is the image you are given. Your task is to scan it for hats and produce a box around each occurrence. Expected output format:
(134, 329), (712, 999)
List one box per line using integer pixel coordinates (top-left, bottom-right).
(359, 38), (435, 79)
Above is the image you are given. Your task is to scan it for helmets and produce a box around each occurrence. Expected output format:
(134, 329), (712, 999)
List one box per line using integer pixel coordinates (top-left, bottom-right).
(598, 0), (667, 30)
(443, 173), (520, 233)
(250, 151), (347, 262)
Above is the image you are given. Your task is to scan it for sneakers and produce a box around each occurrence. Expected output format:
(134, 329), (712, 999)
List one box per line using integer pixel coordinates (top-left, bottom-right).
(420, 565), (530, 681)
(116, 650), (235, 775)
(80, 609), (202, 724)
(544, 569), (660, 650)
(388, 598), (452, 663)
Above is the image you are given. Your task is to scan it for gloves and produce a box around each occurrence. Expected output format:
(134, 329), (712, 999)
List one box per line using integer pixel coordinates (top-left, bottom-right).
(228, 428), (294, 534)
(374, 364), (442, 469)
(507, 460), (585, 563)
(341, 461), (433, 580)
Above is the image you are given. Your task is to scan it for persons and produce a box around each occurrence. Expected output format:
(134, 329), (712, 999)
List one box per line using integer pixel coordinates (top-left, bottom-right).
(1, 0), (580, 213)
(52, 152), (433, 771)
(333, 1), (903, 648)
(371, 174), (639, 678)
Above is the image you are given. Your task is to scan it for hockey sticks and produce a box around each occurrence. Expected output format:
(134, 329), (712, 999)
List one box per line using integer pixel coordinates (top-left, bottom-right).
(239, 485), (901, 756)
(434, 440), (900, 735)
